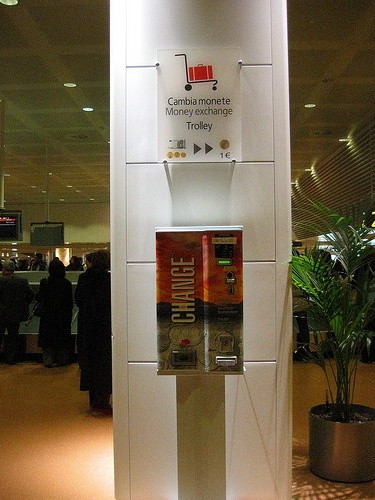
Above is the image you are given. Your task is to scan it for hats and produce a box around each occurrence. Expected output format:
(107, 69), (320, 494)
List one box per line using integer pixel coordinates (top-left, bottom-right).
(0, 259), (15, 270)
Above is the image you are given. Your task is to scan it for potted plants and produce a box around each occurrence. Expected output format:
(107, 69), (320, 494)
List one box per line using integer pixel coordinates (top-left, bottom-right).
(289, 189), (375, 483)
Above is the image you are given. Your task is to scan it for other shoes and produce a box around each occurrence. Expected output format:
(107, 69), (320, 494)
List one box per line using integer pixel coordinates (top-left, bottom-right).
(89, 400), (112, 417)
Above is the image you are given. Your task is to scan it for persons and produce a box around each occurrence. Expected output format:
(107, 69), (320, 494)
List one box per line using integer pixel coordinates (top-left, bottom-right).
(34, 258), (72, 369)
(350, 247), (375, 364)
(75, 250), (112, 416)
(0, 260), (34, 365)
(8, 252), (93, 270)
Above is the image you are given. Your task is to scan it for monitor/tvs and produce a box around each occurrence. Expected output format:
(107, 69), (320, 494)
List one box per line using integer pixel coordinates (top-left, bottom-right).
(29, 222), (64, 246)
(0, 209), (22, 241)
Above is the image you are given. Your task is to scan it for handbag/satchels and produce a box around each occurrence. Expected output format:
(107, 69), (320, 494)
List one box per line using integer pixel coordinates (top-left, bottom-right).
(32, 302), (43, 317)
